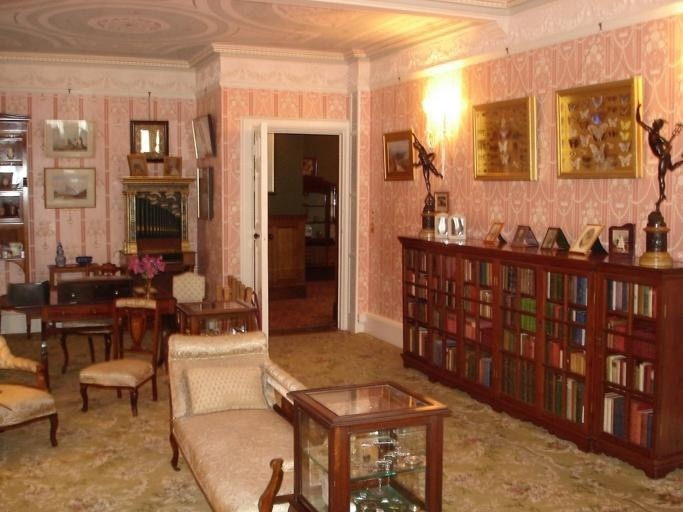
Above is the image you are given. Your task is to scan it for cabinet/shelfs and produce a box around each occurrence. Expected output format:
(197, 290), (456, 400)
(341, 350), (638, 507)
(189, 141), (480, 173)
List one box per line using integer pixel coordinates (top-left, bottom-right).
(0, 115), (35, 340)
(120, 249), (196, 316)
(268, 215), (308, 301)
(302, 182), (340, 267)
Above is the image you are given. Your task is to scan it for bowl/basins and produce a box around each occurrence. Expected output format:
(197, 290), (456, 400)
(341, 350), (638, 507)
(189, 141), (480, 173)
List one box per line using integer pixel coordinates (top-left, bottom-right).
(75, 255), (92, 266)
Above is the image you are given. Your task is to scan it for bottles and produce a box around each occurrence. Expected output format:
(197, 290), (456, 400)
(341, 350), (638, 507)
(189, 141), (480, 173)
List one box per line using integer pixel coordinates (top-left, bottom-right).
(54, 240), (65, 268)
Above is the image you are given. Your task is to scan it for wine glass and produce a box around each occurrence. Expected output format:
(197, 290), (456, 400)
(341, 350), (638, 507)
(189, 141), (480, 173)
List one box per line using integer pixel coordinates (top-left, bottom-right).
(353, 433), (422, 511)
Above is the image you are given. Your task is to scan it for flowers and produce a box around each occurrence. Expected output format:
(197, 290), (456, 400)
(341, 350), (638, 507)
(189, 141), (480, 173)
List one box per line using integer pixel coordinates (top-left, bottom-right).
(128, 255), (167, 279)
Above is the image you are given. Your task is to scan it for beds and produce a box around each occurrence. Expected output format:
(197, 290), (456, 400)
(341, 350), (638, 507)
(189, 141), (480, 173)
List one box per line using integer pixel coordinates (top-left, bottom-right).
(166, 329), (311, 512)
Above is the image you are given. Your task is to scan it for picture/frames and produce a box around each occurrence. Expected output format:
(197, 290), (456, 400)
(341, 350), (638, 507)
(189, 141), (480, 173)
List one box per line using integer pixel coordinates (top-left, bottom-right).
(471, 96), (538, 182)
(382, 128), (417, 181)
(485, 223), (635, 257)
(555, 76), (645, 180)
(434, 191), (449, 213)
(44, 114), (216, 221)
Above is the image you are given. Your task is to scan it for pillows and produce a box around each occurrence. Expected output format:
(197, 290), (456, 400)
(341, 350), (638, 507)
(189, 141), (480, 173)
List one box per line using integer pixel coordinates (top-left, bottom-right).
(168, 355), (270, 418)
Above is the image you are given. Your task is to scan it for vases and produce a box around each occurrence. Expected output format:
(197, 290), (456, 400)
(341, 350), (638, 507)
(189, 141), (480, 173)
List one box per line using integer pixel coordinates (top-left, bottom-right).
(302, 152), (320, 182)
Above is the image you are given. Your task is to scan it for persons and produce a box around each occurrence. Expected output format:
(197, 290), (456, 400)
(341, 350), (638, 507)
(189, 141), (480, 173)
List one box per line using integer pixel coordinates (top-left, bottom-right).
(636, 102), (682, 208)
(407, 131), (442, 192)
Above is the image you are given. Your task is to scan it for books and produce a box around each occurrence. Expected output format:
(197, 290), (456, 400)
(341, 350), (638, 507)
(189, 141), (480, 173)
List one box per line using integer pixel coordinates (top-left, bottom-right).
(603, 279), (656, 449)
(406, 250), (587, 423)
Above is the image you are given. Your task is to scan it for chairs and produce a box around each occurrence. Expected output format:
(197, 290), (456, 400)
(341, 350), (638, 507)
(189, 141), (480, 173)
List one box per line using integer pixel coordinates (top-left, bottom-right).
(0, 276), (160, 448)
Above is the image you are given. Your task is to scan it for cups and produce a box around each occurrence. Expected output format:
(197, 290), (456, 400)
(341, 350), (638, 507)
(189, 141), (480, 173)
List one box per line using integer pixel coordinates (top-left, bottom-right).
(0, 241), (24, 259)
(0, 206), (16, 217)
(6, 147), (15, 160)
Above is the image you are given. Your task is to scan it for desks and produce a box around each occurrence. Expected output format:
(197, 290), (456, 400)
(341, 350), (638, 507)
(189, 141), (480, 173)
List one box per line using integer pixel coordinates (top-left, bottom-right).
(48, 264), (99, 338)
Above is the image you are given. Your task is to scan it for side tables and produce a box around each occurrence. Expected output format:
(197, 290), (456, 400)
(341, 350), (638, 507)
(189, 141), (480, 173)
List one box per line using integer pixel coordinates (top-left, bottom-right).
(286, 382), (452, 512)
(176, 302), (256, 335)
